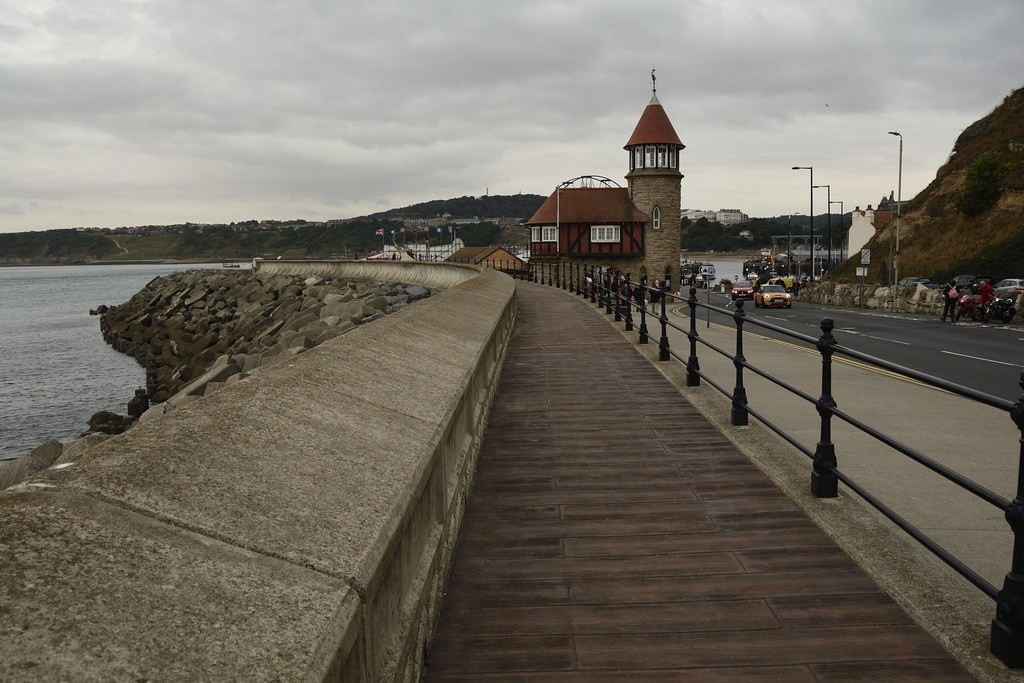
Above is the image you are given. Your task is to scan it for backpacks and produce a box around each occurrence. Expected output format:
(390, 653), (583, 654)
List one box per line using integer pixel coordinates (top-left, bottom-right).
(947, 285), (958, 298)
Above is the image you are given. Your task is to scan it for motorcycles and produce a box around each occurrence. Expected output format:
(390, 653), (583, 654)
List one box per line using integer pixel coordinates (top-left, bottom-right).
(956, 295), (1017, 324)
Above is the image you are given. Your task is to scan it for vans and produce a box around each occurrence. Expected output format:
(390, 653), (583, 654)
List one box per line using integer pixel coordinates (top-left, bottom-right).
(760, 278), (794, 293)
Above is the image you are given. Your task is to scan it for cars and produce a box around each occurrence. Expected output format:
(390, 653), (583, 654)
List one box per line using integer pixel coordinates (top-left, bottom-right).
(899, 275), (1024, 294)
(754, 285), (792, 309)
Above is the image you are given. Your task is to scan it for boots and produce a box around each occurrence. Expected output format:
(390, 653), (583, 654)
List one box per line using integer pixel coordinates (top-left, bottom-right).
(951, 313), (955, 322)
(940, 310), (948, 321)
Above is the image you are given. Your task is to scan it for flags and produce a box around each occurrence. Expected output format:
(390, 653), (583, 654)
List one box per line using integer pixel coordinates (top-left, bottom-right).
(400, 228), (404, 232)
(448, 226), (452, 232)
(424, 227), (429, 231)
(376, 229), (384, 235)
(437, 228), (442, 232)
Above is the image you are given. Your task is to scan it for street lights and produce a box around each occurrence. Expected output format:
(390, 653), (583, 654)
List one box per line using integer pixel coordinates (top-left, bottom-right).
(829, 201), (843, 263)
(812, 185), (831, 271)
(887, 132), (903, 313)
(792, 166), (813, 281)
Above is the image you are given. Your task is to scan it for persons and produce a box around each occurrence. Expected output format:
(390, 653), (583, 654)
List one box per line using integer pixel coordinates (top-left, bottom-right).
(583, 270), (593, 292)
(940, 280), (960, 322)
(979, 281), (993, 321)
(649, 280), (659, 315)
(354, 254), (358, 260)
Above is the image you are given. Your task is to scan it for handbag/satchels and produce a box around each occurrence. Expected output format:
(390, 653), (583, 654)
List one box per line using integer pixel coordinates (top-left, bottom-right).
(644, 299), (648, 306)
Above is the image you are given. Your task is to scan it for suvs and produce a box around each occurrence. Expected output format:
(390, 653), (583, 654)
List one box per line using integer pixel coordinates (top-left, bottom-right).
(731, 281), (754, 301)
